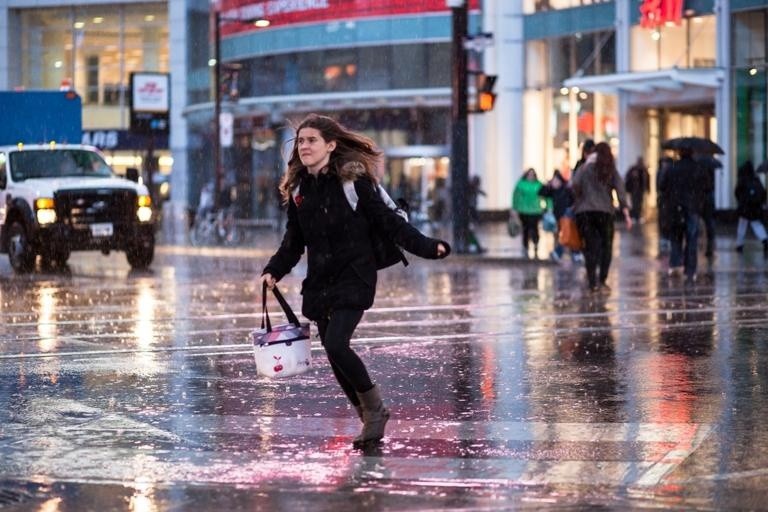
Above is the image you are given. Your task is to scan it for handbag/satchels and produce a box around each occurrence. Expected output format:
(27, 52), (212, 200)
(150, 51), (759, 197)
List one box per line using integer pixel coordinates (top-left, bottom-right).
(509, 216), (519, 237)
(542, 212), (558, 231)
(251, 280), (313, 381)
(557, 215), (583, 253)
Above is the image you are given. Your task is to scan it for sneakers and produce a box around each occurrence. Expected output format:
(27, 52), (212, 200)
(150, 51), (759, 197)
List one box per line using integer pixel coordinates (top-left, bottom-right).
(551, 250), (559, 265)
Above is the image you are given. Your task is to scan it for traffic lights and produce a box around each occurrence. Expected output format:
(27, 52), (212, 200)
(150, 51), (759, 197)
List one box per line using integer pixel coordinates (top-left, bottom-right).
(476, 75), (498, 113)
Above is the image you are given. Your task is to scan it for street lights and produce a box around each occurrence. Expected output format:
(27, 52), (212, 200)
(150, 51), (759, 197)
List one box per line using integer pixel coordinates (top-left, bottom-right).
(209, 10), (272, 245)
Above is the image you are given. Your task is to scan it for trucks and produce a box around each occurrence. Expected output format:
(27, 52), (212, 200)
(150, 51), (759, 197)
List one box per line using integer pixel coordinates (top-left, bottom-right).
(0, 91), (157, 274)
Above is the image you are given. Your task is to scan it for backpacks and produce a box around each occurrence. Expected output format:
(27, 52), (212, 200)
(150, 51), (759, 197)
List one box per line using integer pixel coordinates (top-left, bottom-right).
(292, 169), (411, 270)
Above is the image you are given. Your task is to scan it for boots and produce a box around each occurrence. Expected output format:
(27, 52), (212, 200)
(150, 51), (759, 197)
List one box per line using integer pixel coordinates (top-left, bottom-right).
(352, 384), (391, 447)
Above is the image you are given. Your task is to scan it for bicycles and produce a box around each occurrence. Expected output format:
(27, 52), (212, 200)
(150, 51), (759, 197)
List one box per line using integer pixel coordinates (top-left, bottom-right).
(188, 185), (247, 247)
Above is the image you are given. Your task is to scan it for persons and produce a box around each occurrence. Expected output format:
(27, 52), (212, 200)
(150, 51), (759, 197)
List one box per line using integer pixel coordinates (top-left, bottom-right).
(254, 113), (450, 447)
